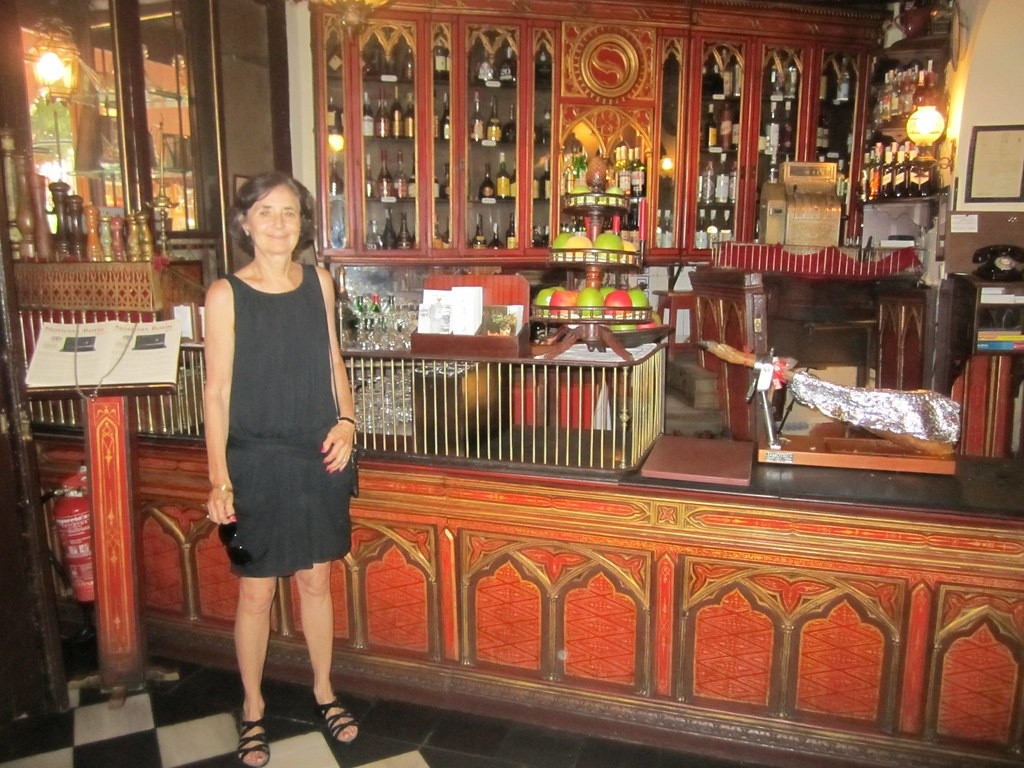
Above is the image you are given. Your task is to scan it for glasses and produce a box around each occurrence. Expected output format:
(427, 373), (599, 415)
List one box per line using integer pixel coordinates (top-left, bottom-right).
(217, 514), (269, 567)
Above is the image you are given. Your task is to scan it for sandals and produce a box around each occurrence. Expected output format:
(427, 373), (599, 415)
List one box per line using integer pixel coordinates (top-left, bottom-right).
(313, 694), (360, 746)
(238, 711), (270, 768)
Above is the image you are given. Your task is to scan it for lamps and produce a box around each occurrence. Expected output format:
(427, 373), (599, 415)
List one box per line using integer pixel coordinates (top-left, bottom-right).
(905, 82), (944, 166)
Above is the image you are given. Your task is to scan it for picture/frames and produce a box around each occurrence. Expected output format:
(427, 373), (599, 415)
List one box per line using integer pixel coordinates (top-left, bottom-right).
(964, 124), (1024, 204)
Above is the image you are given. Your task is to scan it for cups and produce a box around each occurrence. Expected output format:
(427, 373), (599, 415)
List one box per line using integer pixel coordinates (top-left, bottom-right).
(894, 8), (929, 39)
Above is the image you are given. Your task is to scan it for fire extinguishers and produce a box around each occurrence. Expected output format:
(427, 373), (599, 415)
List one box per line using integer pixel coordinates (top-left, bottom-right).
(41, 461), (95, 602)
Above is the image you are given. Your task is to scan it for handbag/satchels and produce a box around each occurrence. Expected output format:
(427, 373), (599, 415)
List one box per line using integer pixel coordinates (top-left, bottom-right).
(350, 445), (359, 497)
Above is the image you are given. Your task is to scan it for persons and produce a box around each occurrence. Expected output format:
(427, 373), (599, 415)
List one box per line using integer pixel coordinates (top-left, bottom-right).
(204, 172), (359, 768)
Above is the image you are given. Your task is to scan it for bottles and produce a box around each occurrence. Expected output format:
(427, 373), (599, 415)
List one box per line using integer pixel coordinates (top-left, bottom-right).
(661, 209), (672, 248)
(858, 59), (943, 202)
(883, 2), (906, 48)
(330, 40), (552, 248)
(655, 210), (662, 248)
(695, 63), (846, 249)
(561, 143), (646, 250)
(333, 265), (400, 350)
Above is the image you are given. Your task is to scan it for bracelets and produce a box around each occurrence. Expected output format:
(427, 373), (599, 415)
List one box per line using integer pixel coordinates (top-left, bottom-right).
(212, 484), (232, 492)
(339, 417), (354, 424)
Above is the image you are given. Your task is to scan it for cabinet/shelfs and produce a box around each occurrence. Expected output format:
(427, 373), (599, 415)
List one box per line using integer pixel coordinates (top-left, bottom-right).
(305, 0), (883, 248)
(950, 271), (1024, 459)
